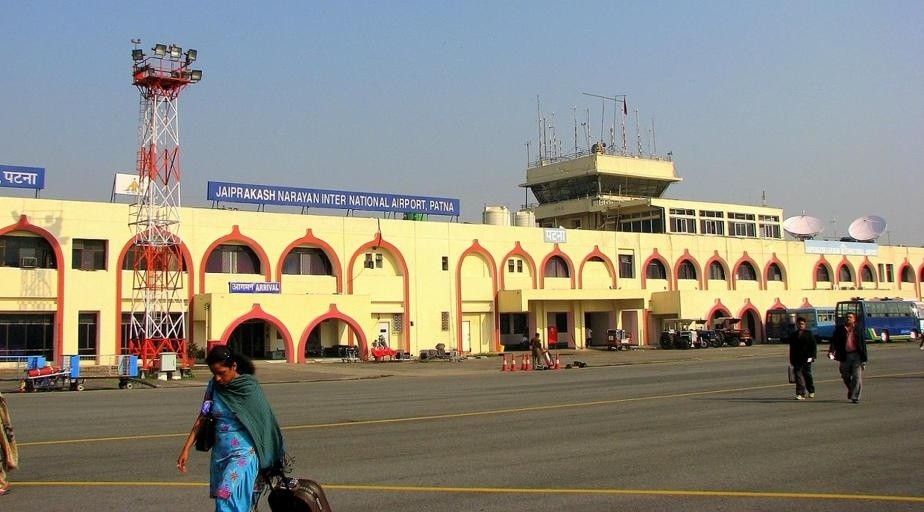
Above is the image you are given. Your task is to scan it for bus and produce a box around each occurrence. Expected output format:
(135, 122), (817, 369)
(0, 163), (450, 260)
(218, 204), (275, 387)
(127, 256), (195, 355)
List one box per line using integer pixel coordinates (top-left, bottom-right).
(765, 299), (921, 342)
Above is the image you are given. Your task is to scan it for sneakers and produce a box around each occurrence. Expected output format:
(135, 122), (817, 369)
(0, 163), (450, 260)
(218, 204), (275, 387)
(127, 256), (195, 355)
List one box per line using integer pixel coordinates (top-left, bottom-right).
(809, 393), (815, 398)
(793, 395), (805, 400)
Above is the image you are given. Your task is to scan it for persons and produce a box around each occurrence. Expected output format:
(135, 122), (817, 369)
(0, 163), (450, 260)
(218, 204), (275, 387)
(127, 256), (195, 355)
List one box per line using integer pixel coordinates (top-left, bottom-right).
(828, 312), (867, 403)
(520, 335), (528, 346)
(585, 327), (592, 348)
(177, 345), (285, 512)
(530, 333), (541, 369)
(790, 317), (817, 400)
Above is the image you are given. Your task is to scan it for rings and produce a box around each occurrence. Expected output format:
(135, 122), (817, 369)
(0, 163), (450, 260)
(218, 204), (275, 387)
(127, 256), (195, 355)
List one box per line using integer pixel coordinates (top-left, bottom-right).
(177, 464), (179, 467)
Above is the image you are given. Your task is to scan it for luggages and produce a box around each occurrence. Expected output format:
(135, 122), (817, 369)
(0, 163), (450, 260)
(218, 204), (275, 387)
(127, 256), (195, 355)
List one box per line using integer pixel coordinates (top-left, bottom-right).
(267, 472), (333, 512)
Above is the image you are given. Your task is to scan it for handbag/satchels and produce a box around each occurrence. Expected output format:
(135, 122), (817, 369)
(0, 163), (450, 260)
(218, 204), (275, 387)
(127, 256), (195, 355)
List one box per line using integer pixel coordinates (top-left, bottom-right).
(788, 367), (796, 383)
(195, 417), (214, 451)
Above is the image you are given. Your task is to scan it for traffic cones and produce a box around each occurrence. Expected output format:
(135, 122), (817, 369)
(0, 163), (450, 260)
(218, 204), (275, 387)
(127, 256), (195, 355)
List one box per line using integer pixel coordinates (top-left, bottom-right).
(501, 352), (561, 370)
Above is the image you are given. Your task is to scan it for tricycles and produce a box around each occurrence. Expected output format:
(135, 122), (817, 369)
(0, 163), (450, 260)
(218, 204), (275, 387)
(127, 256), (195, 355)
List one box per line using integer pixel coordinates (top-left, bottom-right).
(607, 328), (631, 350)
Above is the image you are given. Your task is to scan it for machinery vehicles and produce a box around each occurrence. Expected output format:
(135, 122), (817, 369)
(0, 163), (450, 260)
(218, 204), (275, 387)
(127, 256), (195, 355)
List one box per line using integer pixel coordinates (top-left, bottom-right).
(657, 315), (753, 352)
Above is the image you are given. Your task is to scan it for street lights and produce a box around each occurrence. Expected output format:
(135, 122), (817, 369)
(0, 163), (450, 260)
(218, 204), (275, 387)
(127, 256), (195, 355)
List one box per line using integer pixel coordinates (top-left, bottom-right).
(126, 41), (204, 377)
